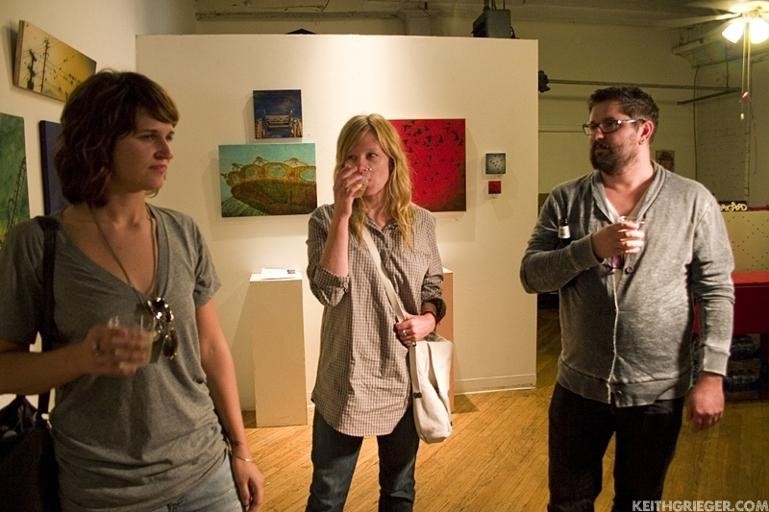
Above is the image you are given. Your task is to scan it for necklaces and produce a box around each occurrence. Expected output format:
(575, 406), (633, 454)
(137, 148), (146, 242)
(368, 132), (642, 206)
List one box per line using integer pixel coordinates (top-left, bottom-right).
(87, 200), (179, 361)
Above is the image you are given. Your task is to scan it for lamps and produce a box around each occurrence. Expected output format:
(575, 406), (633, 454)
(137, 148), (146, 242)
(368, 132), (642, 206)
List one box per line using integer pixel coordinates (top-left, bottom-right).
(721, 12), (769, 45)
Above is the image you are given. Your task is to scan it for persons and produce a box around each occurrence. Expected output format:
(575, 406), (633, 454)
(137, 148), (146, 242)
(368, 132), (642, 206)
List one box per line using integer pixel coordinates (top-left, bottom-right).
(305, 112), (447, 512)
(1, 71), (266, 512)
(520, 86), (734, 511)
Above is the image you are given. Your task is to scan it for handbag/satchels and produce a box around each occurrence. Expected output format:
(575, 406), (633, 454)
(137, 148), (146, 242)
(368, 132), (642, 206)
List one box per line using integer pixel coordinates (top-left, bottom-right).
(0, 394), (61, 512)
(407, 332), (454, 445)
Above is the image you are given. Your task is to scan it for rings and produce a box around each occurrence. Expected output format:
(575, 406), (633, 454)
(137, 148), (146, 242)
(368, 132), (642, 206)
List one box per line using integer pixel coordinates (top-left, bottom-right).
(401, 330), (407, 337)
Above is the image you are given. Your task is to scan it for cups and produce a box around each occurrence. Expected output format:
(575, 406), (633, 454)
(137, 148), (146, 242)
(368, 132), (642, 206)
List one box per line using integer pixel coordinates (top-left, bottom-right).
(343, 159), (375, 200)
(616, 214), (646, 244)
(104, 313), (155, 371)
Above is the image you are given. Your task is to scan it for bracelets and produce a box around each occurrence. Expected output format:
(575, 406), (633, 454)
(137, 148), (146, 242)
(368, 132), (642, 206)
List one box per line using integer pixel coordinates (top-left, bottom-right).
(232, 451), (259, 464)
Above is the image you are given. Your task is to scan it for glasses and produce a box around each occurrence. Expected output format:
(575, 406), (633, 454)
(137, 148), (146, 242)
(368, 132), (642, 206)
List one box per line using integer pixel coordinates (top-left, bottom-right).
(582, 119), (638, 137)
(145, 296), (179, 360)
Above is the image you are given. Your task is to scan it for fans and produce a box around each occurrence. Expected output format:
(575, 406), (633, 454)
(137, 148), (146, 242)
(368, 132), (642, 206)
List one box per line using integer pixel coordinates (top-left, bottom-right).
(670, 0), (769, 30)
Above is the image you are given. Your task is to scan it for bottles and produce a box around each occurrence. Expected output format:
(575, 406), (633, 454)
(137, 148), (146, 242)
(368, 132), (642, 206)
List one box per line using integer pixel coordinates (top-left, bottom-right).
(554, 216), (577, 289)
